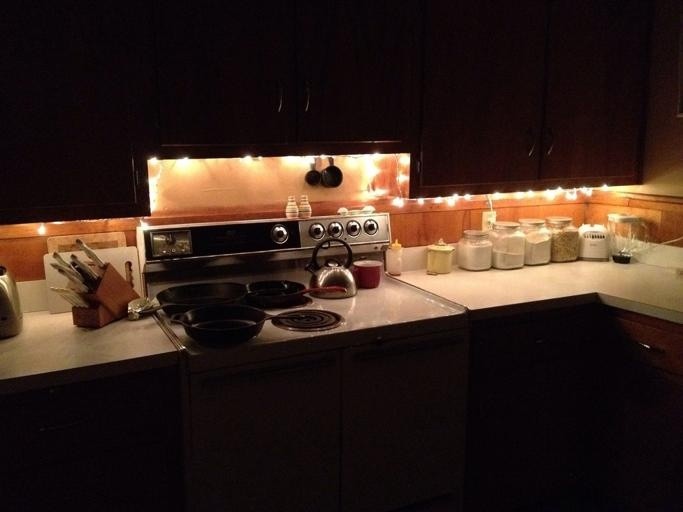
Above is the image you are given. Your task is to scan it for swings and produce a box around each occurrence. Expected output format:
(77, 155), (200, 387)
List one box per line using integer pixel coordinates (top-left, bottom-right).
(580, 212), (643, 263)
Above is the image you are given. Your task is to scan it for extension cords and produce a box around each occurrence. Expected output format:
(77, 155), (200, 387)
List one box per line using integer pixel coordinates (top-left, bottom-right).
(0, 264), (23, 340)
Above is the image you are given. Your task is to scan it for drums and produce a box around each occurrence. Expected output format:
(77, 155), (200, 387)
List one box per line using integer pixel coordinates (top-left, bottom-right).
(47, 238), (104, 287)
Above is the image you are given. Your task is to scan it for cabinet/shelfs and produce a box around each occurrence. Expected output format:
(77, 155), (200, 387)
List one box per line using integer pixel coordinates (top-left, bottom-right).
(388, 239), (402, 276)
(424, 216), (580, 276)
(182, 328), (472, 512)
(0, 0), (651, 225)
(285, 195), (311, 220)
(0, 366), (181, 512)
(463, 303), (596, 512)
(596, 304), (683, 512)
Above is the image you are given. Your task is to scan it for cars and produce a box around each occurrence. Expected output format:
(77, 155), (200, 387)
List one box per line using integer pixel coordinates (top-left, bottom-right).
(352, 259), (383, 291)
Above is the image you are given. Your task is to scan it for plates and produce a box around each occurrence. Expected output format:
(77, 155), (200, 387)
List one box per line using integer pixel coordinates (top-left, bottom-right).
(596, 304), (683, 512)
(182, 328), (472, 512)
(463, 303), (596, 512)
(0, 366), (181, 512)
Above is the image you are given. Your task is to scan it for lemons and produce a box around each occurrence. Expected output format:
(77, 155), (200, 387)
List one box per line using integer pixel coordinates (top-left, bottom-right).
(302, 238), (358, 300)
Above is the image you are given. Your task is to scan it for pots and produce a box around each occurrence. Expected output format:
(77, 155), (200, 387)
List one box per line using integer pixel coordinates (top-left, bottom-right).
(139, 281), (246, 318)
(303, 155), (321, 187)
(321, 156), (344, 189)
(169, 305), (306, 349)
(245, 278), (347, 303)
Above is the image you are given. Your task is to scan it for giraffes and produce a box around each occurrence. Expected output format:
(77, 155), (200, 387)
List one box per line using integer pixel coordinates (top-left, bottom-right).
(134, 214), (469, 352)
